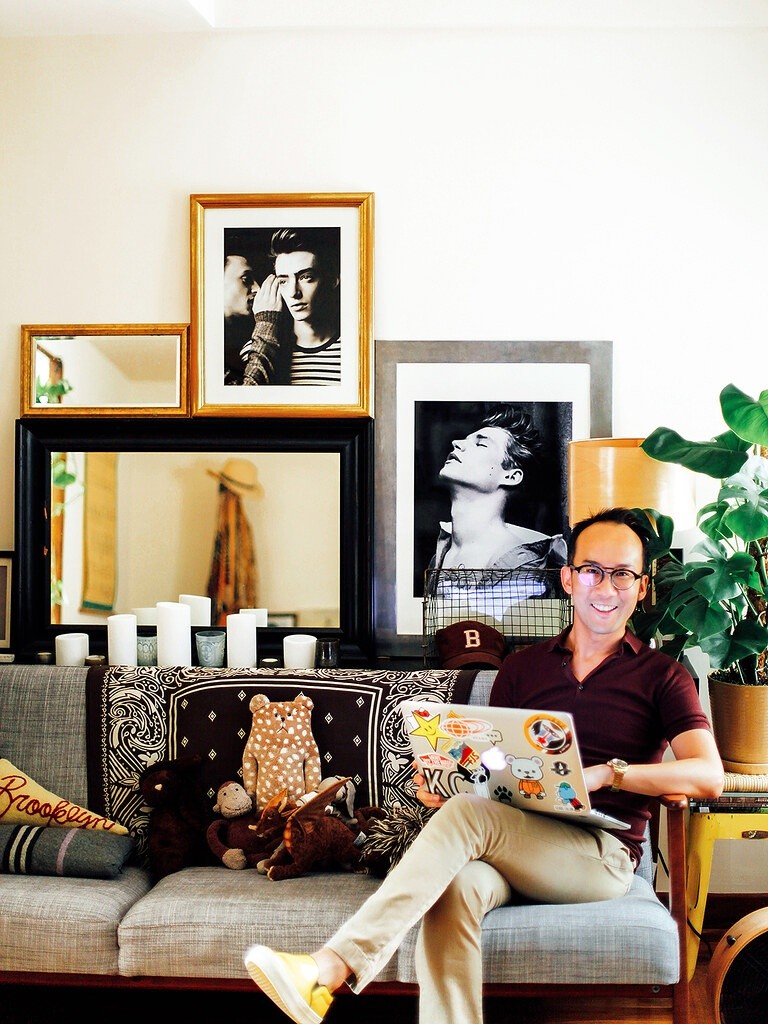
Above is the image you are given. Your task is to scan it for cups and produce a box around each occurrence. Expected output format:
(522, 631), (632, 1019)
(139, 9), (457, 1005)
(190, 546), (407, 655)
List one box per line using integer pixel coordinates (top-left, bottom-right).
(195, 628), (226, 668)
(137, 634), (158, 667)
(315, 636), (340, 669)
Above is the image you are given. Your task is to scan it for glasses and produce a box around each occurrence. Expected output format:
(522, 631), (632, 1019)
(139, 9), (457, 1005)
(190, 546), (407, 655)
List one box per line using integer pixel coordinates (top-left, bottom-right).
(569, 563), (646, 591)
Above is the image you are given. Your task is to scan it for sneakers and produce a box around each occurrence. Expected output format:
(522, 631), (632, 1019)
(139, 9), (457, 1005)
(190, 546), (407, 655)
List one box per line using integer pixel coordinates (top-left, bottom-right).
(244, 941), (335, 1024)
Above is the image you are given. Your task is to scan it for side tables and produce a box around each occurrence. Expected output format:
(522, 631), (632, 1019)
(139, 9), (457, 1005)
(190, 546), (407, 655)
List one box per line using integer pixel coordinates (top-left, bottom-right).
(687, 791), (768, 978)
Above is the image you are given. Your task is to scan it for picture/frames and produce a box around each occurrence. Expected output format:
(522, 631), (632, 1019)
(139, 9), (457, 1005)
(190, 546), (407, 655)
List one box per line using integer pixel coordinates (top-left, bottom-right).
(0, 550), (15, 655)
(373, 340), (612, 658)
(191, 192), (376, 417)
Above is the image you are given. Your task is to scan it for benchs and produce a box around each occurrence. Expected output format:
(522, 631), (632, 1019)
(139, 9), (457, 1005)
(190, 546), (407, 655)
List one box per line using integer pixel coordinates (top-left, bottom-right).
(0, 663), (689, 1024)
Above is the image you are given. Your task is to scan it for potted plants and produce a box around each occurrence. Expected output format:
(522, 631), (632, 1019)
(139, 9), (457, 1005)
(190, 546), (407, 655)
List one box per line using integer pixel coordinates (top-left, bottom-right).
(630, 384), (768, 765)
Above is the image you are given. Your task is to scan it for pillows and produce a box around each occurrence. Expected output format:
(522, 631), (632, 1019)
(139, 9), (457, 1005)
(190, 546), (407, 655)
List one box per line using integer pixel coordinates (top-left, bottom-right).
(0, 823), (134, 878)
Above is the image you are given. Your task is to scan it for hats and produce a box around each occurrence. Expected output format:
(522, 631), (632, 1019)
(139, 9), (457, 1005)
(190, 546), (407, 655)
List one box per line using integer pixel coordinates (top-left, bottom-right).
(206, 458), (265, 501)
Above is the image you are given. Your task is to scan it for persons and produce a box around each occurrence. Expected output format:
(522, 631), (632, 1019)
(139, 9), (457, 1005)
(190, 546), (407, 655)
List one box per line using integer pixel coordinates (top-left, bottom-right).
(240, 229), (341, 388)
(245, 508), (726, 1024)
(427, 408), (568, 613)
(223, 253), (283, 386)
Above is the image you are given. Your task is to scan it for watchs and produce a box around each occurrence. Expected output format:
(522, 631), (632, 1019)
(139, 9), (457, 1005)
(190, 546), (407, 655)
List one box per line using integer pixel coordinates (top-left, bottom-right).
(607, 759), (629, 793)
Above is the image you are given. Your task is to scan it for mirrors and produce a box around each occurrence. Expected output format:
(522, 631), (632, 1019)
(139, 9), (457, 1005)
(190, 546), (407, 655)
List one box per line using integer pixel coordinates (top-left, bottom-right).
(14, 415), (374, 672)
(21, 323), (189, 418)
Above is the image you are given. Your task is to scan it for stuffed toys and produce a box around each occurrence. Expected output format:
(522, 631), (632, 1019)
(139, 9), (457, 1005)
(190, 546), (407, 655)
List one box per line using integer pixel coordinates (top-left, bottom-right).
(138, 760), (390, 881)
